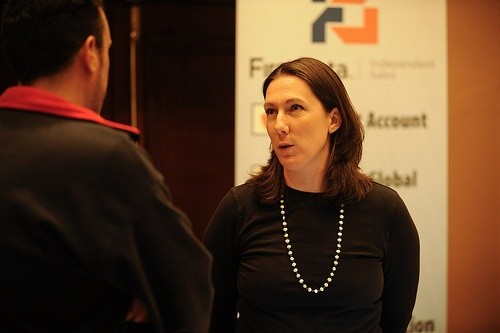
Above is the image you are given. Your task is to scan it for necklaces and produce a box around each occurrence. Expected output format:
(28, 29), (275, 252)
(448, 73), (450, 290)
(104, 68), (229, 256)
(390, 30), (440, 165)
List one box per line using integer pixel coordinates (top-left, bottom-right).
(280, 178), (344, 294)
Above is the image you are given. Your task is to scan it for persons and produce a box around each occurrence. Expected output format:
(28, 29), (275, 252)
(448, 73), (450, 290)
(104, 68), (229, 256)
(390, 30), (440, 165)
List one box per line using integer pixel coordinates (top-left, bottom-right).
(0, 0), (214, 330)
(202, 57), (421, 331)
